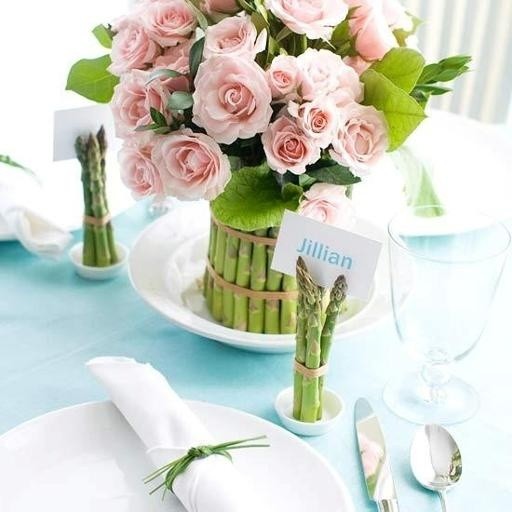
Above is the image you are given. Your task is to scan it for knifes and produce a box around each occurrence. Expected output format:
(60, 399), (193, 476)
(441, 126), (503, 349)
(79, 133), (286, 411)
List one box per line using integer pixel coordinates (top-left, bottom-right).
(355, 396), (401, 512)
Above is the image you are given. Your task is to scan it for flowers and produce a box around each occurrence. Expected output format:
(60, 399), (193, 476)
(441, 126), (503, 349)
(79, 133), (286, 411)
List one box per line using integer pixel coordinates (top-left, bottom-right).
(61, 0), (474, 332)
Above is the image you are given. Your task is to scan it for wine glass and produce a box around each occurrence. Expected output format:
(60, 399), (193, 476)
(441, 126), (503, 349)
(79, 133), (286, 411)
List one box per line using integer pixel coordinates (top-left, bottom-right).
(387, 206), (511, 428)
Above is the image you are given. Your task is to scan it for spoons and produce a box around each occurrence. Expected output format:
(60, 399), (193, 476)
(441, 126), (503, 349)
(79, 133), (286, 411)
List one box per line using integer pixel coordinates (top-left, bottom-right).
(409, 424), (464, 511)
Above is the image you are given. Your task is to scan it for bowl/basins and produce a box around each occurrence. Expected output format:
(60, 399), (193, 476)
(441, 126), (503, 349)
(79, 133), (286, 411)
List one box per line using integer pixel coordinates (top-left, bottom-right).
(70, 240), (127, 282)
(128, 198), (392, 355)
(273, 383), (342, 436)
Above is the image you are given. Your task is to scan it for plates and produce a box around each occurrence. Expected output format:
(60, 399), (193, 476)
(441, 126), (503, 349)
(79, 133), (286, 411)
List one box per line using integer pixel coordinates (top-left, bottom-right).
(0, 396), (356, 511)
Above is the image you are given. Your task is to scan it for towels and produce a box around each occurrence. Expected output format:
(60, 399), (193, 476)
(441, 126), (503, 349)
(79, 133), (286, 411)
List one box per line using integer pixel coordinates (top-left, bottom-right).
(0, 146), (77, 262)
(88, 355), (263, 512)
